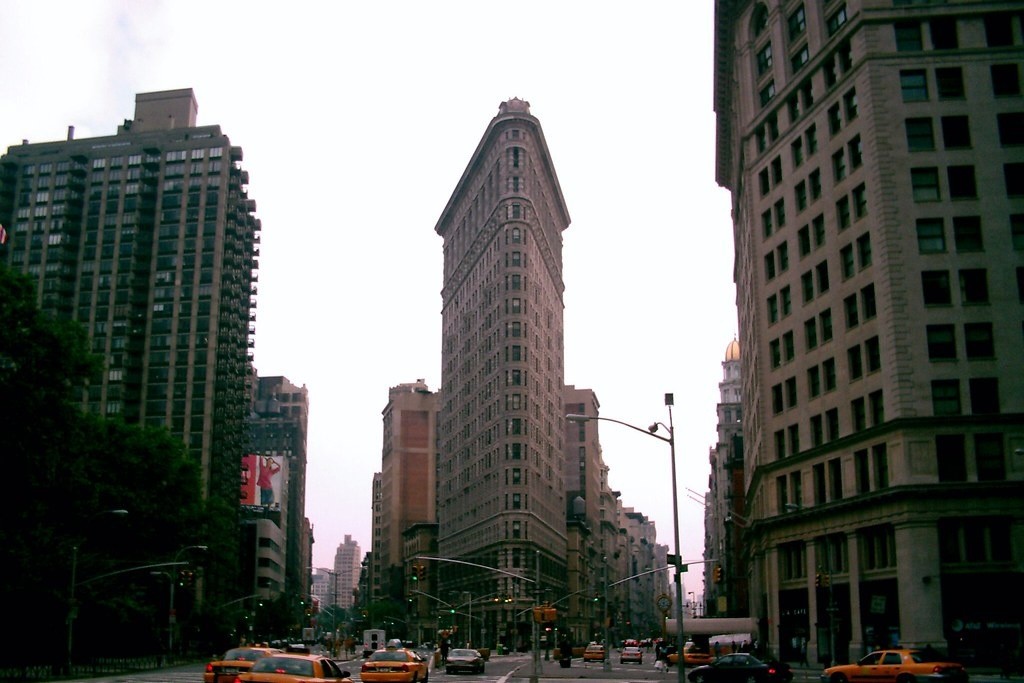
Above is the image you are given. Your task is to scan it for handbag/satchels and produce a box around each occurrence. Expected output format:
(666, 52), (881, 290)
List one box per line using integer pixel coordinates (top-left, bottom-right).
(654, 661), (663, 670)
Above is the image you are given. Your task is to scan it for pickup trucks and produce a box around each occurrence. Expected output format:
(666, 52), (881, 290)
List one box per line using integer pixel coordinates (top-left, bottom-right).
(820, 649), (969, 683)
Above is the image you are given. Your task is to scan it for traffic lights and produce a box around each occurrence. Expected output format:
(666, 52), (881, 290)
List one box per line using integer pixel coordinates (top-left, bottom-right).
(594, 593), (598, 602)
(450, 609), (455, 614)
(408, 597), (413, 609)
(411, 566), (418, 581)
(815, 574), (820, 588)
(625, 619), (632, 626)
(187, 572), (196, 588)
(714, 565), (722, 583)
(419, 566), (426, 582)
(176, 572), (185, 591)
(823, 575), (830, 588)
(494, 597), (499, 603)
(504, 598), (513, 603)
(554, 626), (558, 631)
(532, 610), (539, 624)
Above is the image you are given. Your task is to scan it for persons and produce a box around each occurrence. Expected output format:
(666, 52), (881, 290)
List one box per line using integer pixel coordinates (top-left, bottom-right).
(257, 455), (281, 508)
(732, 641), (736, 653)
(655, 642), (669, 673)
(741, 640), (748, 652)
(800, 637), (809, 671)
(714, 641), (720, 660)
(753, 640), (760, 658)
(440, 640), (448, 661)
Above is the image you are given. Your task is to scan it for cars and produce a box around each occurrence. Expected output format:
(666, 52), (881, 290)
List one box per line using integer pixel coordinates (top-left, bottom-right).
(583, 645), (606, 662)
(445, 648), (486, 675)
(623, 637), (662, 648)
(619, 647), (644, 665)
(203, 646), (286, 683)
(360, 638), (429, 683)
(233, 652), (355, 683)
(687, 652), (794, 683)
(666, 646), (715, 667)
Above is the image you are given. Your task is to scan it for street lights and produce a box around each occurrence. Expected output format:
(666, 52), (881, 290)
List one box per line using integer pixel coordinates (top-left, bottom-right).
(64, 509), (129, 674)
(168, 545), (209, 664)
(565, 393), (688, 683)
(306, 566), (368, 657)
